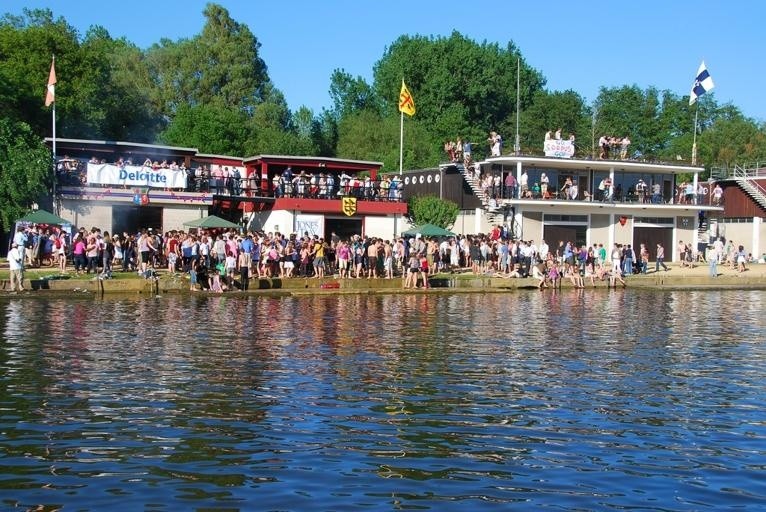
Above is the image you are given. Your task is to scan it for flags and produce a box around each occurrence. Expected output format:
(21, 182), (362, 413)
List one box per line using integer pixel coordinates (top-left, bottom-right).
(688, 63), (715, 106)
(46, 58), (56, 107)
(397, 84), (417, 118)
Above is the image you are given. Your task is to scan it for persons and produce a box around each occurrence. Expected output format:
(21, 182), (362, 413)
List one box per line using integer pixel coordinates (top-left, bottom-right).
(679, 236), (752, 276)
(6, 221), (668, 290)
(447, 130), (723, 208)
(56, 155), (404, 202)
